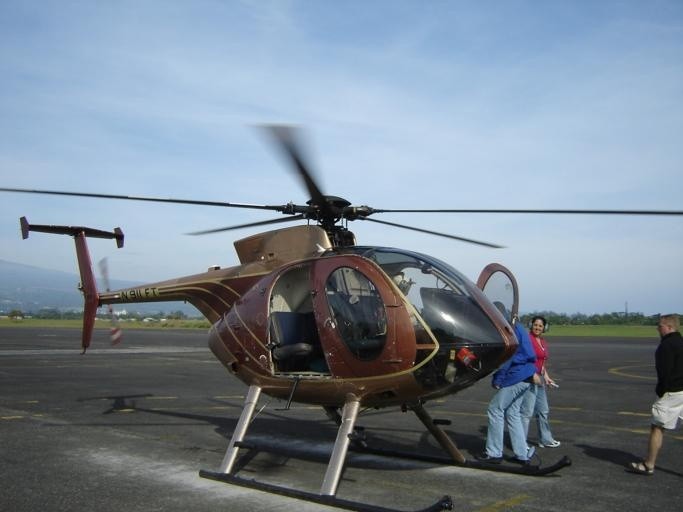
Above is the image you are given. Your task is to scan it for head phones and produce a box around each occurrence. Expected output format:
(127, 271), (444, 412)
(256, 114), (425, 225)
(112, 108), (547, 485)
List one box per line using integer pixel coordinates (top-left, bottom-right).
(528, 315), (549, 333)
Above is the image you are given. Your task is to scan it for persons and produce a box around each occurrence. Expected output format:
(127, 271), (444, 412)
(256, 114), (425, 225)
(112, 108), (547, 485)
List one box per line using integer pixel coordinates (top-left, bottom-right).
(472, 301), (537, 468)
(628, 315), (682, 475)
(518, 316), (560, 449)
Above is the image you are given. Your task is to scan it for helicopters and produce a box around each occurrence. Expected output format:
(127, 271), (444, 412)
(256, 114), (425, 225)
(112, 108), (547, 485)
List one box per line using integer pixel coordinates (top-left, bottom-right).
(0, 114), (683, 512)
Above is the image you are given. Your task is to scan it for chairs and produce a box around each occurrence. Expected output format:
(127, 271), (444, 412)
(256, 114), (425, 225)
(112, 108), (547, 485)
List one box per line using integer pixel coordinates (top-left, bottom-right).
(326, 292), (387, 359)
(268, 311), (316, 360)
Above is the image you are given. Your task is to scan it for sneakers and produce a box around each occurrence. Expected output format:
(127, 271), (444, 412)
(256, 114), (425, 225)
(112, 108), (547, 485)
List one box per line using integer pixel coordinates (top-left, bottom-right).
(510, 456), (530, 464)
(539, 440), (560, 449)
(475, 452), (502, 464)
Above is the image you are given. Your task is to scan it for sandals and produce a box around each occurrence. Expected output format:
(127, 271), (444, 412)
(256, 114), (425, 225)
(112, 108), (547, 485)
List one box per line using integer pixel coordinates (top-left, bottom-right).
(630, 462), (654, 475)
